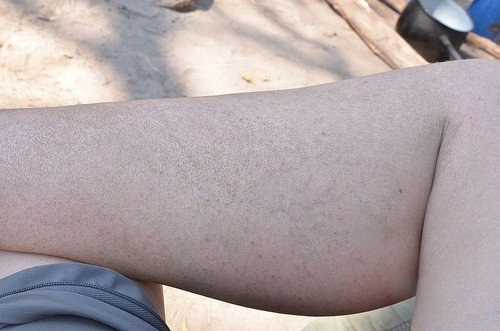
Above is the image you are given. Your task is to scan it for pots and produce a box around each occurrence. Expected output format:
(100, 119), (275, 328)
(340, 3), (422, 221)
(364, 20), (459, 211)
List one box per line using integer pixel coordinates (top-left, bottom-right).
(395, 0), (474, 62)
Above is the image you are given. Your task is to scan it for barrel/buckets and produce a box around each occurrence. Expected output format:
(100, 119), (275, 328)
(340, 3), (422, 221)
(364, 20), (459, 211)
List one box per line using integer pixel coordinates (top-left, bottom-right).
(468, 0), (500, 43)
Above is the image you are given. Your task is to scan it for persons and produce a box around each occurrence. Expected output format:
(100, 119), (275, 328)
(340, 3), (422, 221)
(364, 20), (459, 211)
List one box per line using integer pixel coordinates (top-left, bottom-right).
(1, 60), (500, 331)
